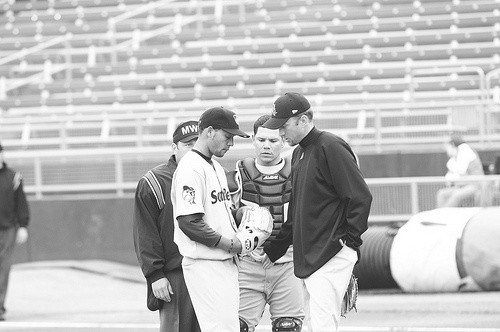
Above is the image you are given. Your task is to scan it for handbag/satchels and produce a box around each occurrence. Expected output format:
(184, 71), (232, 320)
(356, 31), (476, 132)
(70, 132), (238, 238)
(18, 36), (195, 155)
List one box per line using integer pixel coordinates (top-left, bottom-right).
(341, 273), (359, 319)
(147, 282), (163, 311)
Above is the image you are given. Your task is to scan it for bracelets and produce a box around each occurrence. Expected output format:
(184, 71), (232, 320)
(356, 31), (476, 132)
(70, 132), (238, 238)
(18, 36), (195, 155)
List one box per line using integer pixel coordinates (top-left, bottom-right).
(229, 239), (235, 254)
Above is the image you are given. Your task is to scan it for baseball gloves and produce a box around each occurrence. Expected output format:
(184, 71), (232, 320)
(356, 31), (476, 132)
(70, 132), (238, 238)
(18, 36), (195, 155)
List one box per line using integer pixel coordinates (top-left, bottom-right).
(235, 206), (275, 256)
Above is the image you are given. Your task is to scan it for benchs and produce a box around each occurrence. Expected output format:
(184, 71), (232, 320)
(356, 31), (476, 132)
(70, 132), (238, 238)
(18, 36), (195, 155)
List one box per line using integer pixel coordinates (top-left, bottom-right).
(0, 0), (500, 176)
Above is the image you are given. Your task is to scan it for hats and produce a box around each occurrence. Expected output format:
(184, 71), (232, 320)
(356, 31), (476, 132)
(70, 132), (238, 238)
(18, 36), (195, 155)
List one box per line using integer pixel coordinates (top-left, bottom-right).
(172, 120), (200, 144)
(262, 91), (311, 130)
(198, 106), (250, 139)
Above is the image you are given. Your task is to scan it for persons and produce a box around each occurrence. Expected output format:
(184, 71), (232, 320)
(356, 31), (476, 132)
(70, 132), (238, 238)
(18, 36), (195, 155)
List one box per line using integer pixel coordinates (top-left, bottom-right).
(135, 91), (373, 332)
(436, 139), (484, 206)
(0, 146), (29, 319)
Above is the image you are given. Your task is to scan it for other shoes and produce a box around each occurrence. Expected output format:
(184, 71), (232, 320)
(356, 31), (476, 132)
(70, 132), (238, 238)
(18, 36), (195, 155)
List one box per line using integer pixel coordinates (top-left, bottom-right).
(0, 312), (5, 320)
